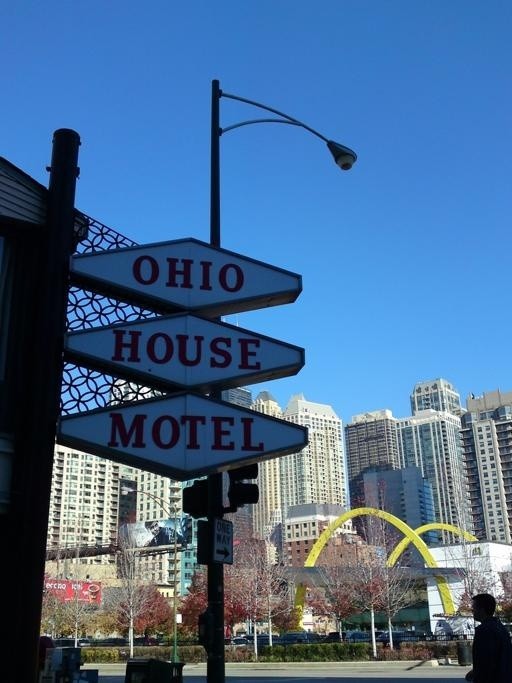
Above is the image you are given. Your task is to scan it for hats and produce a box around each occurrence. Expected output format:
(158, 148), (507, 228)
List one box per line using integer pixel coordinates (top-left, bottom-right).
(229, 629), (432, 644)
(51, 636), (161, 648)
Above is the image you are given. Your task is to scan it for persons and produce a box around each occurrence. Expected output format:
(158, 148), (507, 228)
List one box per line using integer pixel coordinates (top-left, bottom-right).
(140, 520), (186, 547)
(465, 594), (511, 682)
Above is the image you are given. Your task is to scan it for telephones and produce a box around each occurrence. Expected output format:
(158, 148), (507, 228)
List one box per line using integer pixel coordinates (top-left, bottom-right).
(213, 517), (233, 565)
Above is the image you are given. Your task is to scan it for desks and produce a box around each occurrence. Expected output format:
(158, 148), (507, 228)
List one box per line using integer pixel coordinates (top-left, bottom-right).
(210, 89), (357, 675)
(118, 485), (178, 665)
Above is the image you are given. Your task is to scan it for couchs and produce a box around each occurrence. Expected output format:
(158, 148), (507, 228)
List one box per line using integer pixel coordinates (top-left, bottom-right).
(230, 463), (260, 506)
